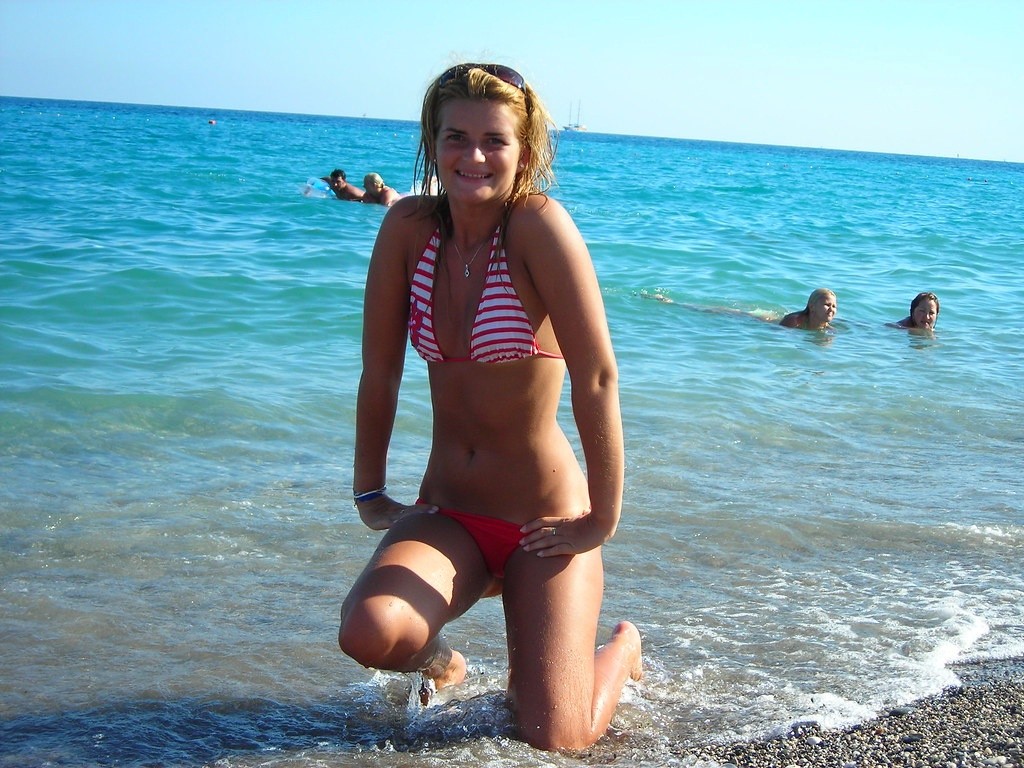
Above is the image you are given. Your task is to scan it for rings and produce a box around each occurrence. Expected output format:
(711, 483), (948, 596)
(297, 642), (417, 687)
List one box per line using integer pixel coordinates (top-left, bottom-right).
(553, 527), (557, 536)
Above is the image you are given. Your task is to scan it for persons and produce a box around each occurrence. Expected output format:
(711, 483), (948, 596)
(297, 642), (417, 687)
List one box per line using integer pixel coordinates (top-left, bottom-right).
(332, 60), (642, 751)
(779, 288), (837, 331)
(884, 292), (940, 333)
(303, 167), (366, 201)
(362, 172), (439, 207)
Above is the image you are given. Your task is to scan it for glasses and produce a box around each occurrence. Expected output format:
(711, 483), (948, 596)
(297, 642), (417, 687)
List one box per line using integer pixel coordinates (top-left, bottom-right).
(437, 63), (526, 96)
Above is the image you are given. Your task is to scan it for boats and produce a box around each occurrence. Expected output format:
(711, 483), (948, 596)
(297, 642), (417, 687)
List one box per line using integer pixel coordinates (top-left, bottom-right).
(563, 99), (587, 131)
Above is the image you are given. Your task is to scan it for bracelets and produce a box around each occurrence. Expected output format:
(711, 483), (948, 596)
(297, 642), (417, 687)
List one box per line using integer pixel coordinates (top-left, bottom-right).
(353, 483), (387, 506)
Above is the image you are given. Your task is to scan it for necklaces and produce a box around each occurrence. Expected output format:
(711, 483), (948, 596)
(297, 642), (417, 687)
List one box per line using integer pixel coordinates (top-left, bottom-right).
(450, 230), (493, 278)
